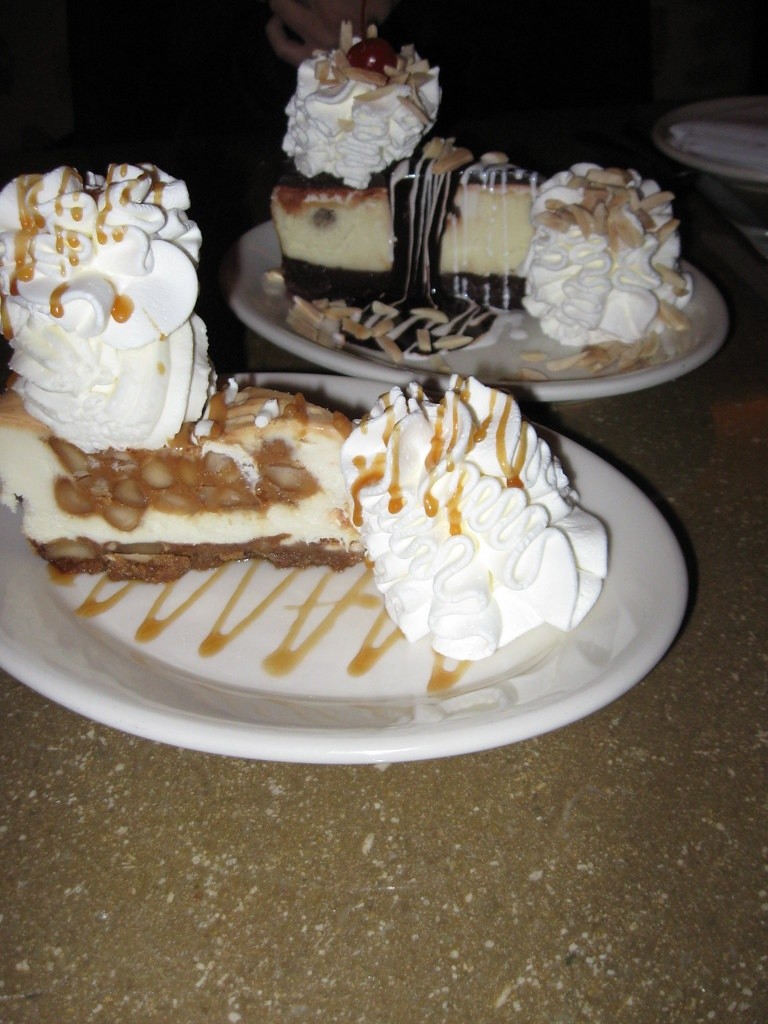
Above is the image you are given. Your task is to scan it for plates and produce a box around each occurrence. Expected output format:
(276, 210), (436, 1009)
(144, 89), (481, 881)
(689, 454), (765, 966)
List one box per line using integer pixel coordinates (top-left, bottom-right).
(655, 95), (768, 181)
(1, 373), (689, 764)
(231, 221), (729, 402)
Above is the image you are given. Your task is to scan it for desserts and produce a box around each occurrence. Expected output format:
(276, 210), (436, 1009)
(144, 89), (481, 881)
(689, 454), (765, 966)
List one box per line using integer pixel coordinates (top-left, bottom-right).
(0, 166), (610, 666)
(272, 32), (690, 348)
